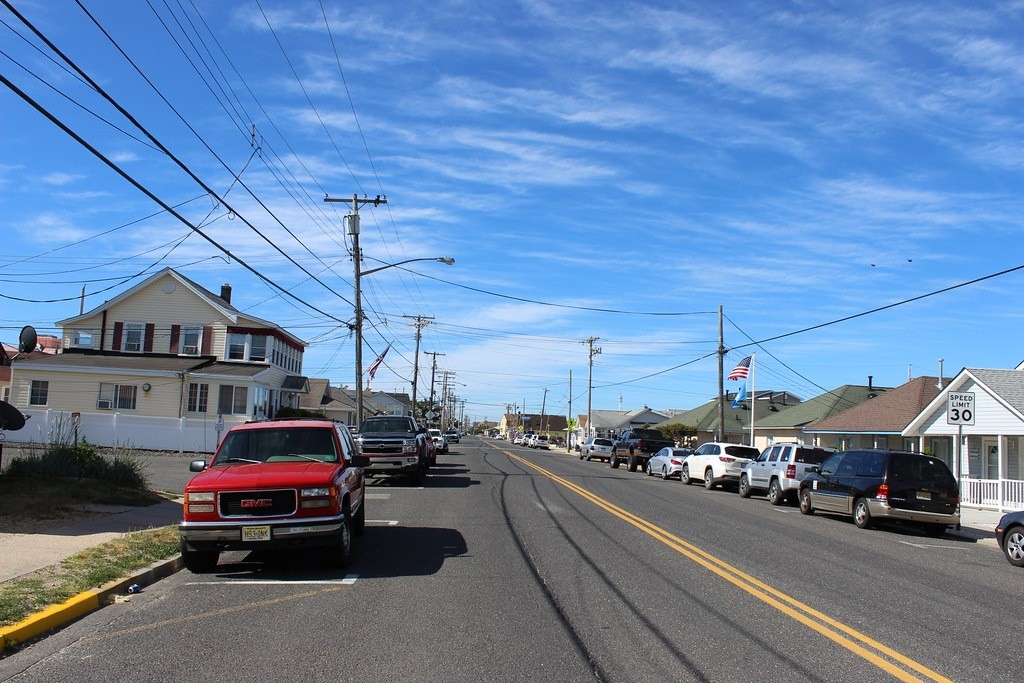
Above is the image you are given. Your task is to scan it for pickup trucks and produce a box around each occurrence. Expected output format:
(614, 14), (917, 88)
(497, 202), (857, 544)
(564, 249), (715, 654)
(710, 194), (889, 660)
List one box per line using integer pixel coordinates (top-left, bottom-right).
(610, 427), (676, 473)
(521, 434), (531, 447)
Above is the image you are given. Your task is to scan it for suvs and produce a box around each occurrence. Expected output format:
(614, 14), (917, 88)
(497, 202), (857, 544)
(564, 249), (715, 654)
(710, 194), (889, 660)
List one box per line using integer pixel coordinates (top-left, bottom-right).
(350, 416), (429, 482)
(444, 431), (459, 443)
(528, 435), (537, 447)
(578, 438), (613, 463)
(179, 417), (367, 573)
(680, 441), (761, 492)
(533, 436), (549, 450)
(739, 441), (838, 506)
(797, 446), (961, 535)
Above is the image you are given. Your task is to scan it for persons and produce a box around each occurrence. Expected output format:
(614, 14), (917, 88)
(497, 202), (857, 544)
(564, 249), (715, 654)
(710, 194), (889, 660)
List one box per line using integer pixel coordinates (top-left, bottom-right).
(675, 440), (692, 448)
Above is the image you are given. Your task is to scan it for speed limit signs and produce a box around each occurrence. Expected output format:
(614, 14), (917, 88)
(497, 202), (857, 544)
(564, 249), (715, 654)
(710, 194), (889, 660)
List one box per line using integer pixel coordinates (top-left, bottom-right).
(947, 390), (975, 425)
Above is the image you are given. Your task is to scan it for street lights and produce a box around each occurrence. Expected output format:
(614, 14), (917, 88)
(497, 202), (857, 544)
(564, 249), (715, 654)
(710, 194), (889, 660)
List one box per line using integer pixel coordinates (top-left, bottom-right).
(430, 378), (466, 410)
(353, 257), (456, 426)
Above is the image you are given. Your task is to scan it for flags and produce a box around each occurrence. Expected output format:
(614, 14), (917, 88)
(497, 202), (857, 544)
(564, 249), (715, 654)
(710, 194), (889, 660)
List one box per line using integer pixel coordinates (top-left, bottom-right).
(731, 381), (746, 408)
(728, 356), (752, 380)
(367, 344), (392, 379)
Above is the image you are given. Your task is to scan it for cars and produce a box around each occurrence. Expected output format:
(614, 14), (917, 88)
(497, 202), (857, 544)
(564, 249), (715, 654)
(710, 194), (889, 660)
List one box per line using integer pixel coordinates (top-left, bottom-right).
(994, 511), (1024, 566)
(429, 429), (445, 452)
(495, 434), (504, 440)
(457, 432), (462, 439)
(645, 446), (695, 480)
(419, 427), (438, 467)
(549, 437), (564, 445)
(514, 436), (523, 445)
